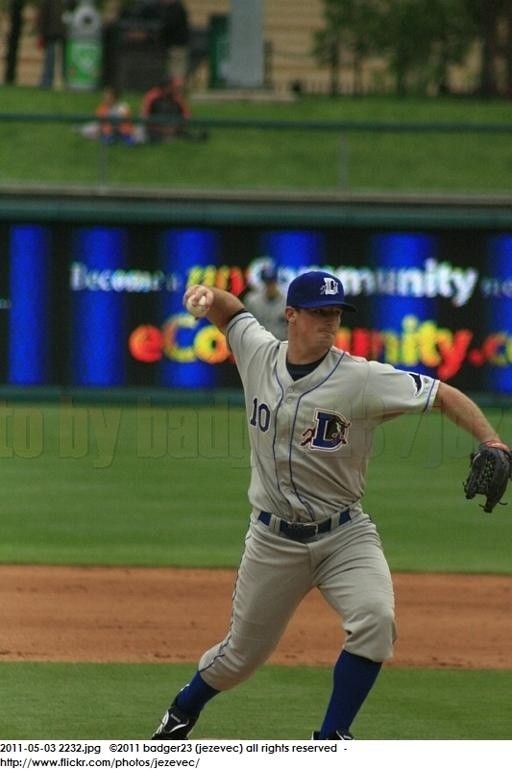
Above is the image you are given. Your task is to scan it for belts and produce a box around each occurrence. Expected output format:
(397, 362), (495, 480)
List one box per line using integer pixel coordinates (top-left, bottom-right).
(254, 506), (352, 544)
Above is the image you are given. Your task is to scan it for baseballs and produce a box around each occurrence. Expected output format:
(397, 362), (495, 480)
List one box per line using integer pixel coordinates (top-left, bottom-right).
(185, 294), (210, 319)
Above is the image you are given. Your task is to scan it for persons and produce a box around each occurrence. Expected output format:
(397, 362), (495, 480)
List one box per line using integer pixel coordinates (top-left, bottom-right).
(154, 272), (510, 737)
(37, 1), (68, 92)
(142, 74), (209, 142)
(97, 83), (143, 147)
(241, 264), (289, 343)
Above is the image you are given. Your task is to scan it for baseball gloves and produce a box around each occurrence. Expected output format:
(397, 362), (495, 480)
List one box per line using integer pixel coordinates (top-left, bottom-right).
(463, 442), (512, 513)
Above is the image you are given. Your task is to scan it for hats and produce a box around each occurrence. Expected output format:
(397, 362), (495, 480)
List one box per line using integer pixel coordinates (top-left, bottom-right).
(285, 269), (359, 313)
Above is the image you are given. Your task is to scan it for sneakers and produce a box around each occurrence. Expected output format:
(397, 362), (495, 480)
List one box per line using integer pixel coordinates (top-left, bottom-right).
(150, 684), (202, 740)
(310, 729), (355, 740)
